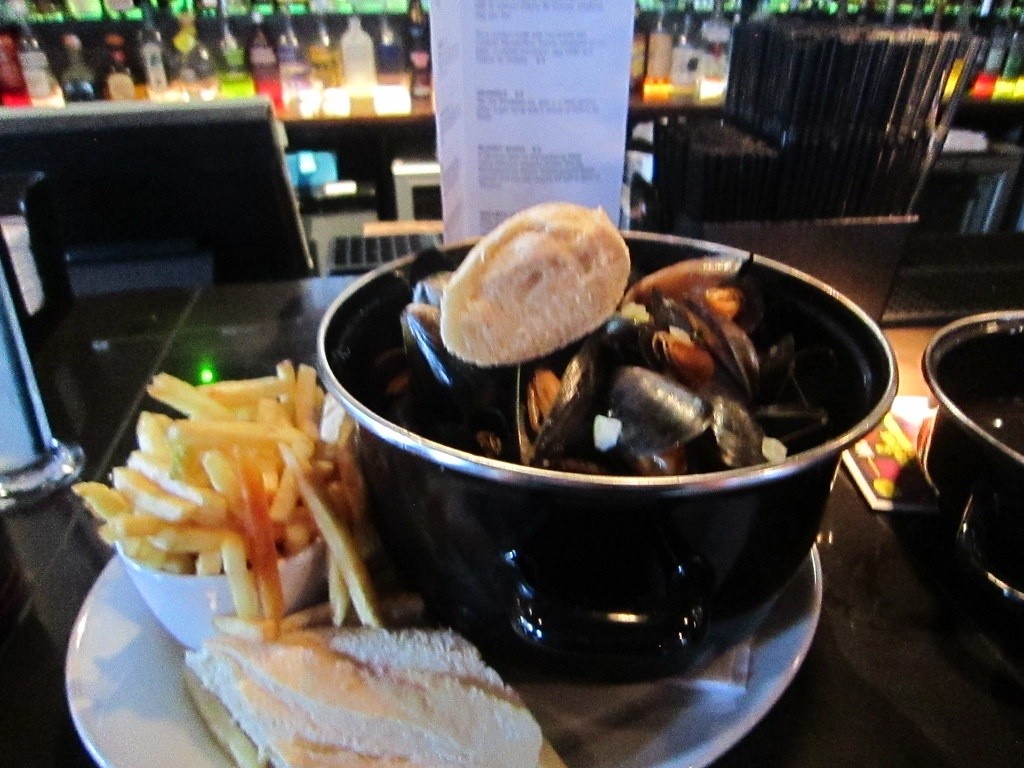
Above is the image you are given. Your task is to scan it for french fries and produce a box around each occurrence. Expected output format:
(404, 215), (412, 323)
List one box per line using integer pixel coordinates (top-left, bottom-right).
(71, 359), (384, 638)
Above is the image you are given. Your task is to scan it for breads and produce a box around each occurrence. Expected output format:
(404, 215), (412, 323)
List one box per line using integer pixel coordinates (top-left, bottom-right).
(438, 201), (631, 366)
(180, 630), (567, 767)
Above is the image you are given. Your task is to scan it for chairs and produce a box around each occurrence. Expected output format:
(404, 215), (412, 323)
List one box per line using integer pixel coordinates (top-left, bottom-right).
(2, 98), (315, 293)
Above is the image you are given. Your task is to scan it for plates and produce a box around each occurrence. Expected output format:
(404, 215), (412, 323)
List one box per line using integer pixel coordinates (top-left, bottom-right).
(65, 540), (825, 768)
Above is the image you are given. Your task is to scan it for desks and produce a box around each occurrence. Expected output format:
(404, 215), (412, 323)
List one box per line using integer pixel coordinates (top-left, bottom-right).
(0, 274), (1024, 768)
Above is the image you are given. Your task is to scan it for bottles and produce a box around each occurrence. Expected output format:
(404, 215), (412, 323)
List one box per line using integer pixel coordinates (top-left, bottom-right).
(629, 1), (741, 107)
(0, 0), (433, 107)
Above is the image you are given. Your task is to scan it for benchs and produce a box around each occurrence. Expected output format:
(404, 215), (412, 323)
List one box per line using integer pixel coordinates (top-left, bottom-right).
(658, 19), (1024, 222)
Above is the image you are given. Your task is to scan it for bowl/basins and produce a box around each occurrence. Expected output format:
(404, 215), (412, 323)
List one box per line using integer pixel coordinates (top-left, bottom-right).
(118, 540), (322, 648)
(923, 310), (1024, 598)
(315, 232), (899, 683)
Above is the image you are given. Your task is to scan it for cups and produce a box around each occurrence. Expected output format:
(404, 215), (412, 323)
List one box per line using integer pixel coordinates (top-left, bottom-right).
(1, 258), (86, 512)
(369, 84), (411, 116)
